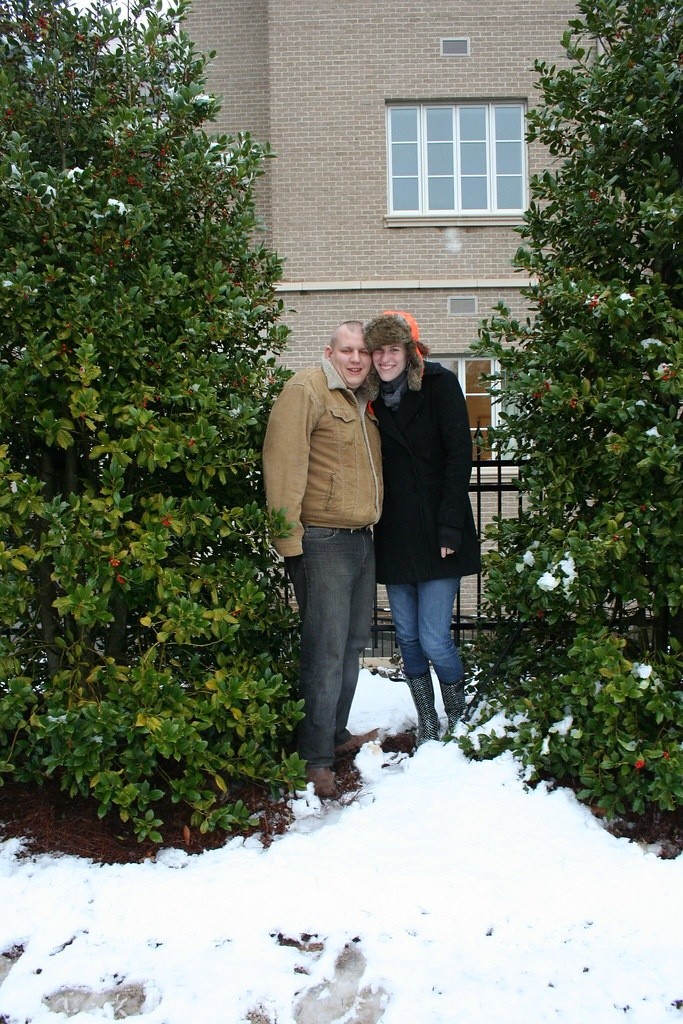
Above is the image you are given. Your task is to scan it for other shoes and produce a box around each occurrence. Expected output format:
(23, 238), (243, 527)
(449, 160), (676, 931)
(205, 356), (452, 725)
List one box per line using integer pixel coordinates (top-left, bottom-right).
(334, 728), (379, 756)
(304, 764), (339, 800)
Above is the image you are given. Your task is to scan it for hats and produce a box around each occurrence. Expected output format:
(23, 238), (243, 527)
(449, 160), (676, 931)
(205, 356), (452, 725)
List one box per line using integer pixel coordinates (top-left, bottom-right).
(363, 310), (431, 401)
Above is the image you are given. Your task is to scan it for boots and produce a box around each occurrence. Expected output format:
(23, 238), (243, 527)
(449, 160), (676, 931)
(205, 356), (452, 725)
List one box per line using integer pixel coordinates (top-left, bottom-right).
(404, 669), (440, 747)
(439, 679), (466, 741)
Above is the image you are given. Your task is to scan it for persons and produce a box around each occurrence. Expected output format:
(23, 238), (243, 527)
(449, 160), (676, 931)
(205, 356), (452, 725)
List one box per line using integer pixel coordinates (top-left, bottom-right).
(261, 321), (385, 799)
(366, 309), (479, 751)
